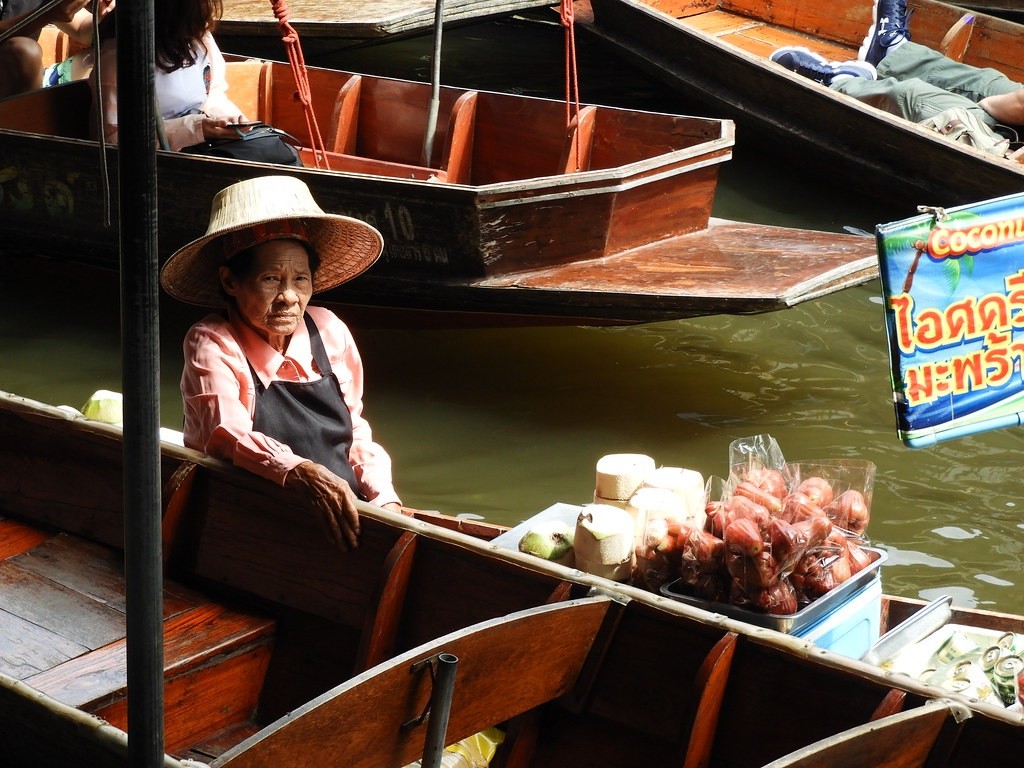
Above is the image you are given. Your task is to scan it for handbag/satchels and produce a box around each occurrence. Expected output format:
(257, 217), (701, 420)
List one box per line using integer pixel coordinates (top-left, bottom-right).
(917, 106), (1010, 158)
(207, 124), (303, 165)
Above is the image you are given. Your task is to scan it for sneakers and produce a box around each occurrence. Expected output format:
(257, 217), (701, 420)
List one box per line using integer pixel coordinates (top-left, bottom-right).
(857, 0), (915, 69)
(769, 46), (878, 87)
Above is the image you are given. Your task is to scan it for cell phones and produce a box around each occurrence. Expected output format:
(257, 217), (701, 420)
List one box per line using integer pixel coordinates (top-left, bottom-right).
(225, 121), (260, 128)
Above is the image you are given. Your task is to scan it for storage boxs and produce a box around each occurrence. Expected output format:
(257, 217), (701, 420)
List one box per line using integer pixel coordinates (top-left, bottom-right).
(659, 547), (889, 667)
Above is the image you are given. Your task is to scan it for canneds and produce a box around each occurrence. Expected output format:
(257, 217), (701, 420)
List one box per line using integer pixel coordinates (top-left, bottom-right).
(888, 630), (1024, 713)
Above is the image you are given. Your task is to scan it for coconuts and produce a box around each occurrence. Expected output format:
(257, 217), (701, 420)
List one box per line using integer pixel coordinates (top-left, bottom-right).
(518, 522), (575, 569)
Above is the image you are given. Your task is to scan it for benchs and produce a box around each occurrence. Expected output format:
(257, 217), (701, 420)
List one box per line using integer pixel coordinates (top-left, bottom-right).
(19, 600), (280, 755)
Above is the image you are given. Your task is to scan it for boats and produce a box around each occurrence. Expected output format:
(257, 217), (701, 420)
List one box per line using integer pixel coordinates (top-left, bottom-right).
(551, 0), (1024, 202)
(0, 386), (1024, 768)
(0, 17), (881, 332)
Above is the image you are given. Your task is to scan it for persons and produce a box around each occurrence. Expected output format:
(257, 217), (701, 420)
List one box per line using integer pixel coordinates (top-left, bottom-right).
(159, 175), (403, 552)
(770, 1), (1024, 165)
(0, 0), (115, 99)
(88, 1), (250, 152)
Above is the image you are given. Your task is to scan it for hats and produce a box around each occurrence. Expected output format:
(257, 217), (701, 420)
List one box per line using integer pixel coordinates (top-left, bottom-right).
(159, 175), (385, 311)
